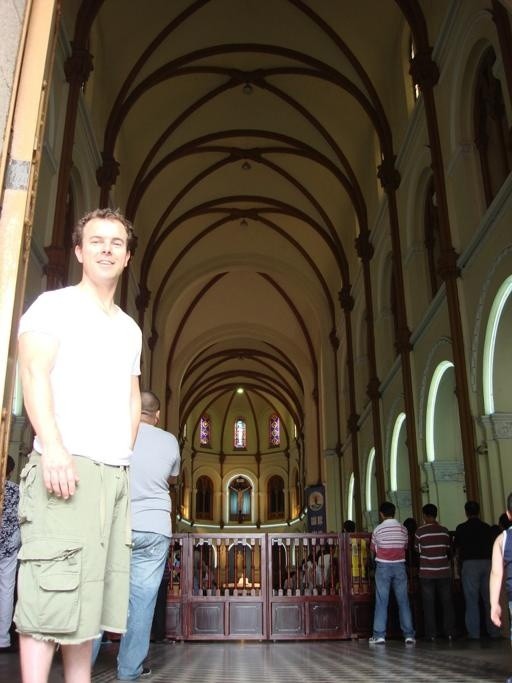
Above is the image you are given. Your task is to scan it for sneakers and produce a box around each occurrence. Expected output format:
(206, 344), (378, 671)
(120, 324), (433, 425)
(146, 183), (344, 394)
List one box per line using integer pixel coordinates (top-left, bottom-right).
(405, 637), (416, 644)
(142, 667), (151, 675)
(369, 643), (385, 652)
(369, 637), (385, 643)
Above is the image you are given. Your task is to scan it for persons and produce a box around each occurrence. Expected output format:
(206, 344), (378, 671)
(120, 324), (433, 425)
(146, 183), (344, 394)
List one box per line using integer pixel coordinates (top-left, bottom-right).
(230, 485), (250, 511)
